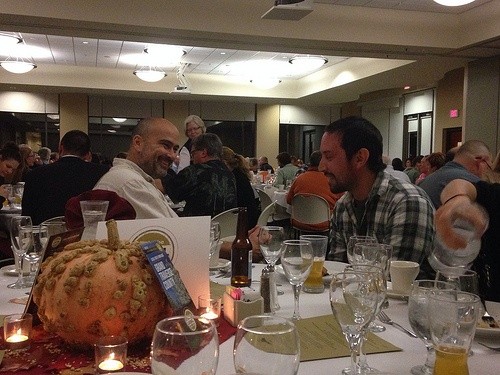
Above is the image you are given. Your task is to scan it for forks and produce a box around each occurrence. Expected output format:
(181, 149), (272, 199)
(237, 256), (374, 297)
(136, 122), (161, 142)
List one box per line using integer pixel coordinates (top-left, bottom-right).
(475, 289), (497, 324)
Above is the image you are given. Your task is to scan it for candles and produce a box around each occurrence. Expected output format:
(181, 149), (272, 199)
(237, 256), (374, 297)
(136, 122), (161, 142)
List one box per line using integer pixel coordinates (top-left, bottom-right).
(93, 334), (128, 375)
(3, 312), (34, 349)
(198, 294), (222, 328)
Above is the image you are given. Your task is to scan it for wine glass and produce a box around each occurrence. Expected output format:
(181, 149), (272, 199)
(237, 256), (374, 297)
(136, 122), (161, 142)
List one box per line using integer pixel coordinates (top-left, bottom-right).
(328, 235), (480, 374)
(1, 182), (64, 287)
(280, 239), (312, 320)
(258, 225), (285, 295)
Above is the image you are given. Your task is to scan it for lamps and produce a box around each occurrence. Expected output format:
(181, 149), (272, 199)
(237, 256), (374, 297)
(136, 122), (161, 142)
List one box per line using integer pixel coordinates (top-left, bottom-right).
(133, 62), (167, 83)
(144, 44), (186, 60)
(0, 52), (39, 74)
(0, 31), (22, 47)
(449, 109), (459, 118)
(288, 54), (330, 71)
(251, 69), (282, 90)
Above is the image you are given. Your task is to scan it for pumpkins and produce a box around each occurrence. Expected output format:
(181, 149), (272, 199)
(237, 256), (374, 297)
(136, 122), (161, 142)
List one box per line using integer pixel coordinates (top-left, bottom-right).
(32, 217), (173, 348)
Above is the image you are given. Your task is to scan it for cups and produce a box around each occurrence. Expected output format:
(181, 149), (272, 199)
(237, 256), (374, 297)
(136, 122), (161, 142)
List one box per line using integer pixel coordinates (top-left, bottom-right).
(389, 260), (420, 295)
(80, 199), (110, 243)
(4, 313), (33, 349)
(233, 315), (301, 375)
(94, 334), (128, 375)
(150, 312), (219, 375)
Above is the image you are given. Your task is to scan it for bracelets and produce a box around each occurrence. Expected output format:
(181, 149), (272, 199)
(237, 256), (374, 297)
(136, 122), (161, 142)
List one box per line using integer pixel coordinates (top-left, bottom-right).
(443, 193), (471, 204)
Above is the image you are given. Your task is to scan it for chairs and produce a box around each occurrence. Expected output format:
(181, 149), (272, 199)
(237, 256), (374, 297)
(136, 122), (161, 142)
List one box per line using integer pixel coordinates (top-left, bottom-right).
(289, 192), (336, 255)
(255, 188), (292, 239)
(210, 207), (241, 256)
(254, 199), (279, 263)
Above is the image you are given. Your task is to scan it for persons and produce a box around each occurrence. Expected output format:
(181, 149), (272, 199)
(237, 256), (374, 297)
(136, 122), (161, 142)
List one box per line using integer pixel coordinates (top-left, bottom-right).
(381, 141), (500, 304)
(316, 116), (438, 286)
(91, 117), (273, 263)
(0, 130), (128, 227)
(153, 115), (347, 231)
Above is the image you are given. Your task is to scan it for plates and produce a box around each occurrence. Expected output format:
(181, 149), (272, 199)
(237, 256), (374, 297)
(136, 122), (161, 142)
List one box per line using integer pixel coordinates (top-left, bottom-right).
(471, 300), (500, 339)
(385, 287), (406, 299)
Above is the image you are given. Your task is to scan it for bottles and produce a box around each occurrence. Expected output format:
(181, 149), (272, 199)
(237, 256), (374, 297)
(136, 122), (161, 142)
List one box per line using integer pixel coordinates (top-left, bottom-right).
(260, 268), (272, 313)
(299, 234), (327, 293)
(266, 264), (280, 311)
(231, 207), (254, 288)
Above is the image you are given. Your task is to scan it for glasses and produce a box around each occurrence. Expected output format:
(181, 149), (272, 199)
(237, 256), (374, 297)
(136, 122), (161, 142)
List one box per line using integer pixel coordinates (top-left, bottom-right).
(28, 154), (35, 158)
(189, 149), (200, 158)
(187, 126), (200, 132)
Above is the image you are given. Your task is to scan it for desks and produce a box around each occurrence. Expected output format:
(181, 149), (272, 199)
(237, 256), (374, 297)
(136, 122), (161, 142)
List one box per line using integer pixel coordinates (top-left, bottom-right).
(271, 190), (300, 241)
(0, 260), (500, 375)
